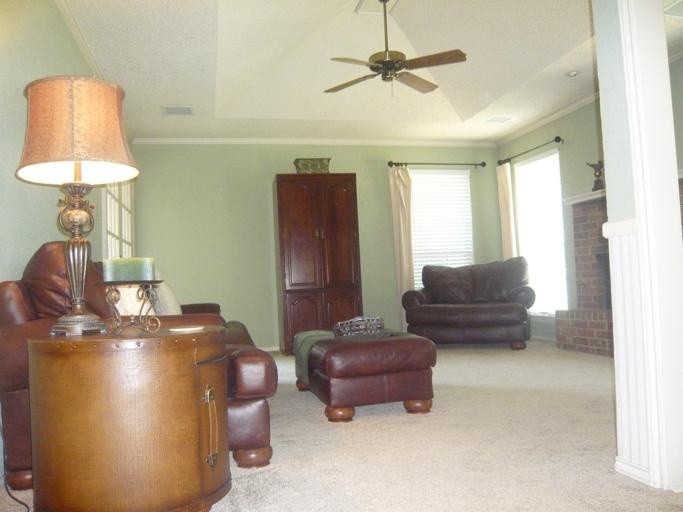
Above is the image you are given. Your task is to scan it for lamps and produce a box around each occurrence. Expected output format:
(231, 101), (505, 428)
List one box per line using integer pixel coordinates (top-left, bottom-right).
(16, 75), (140, 336)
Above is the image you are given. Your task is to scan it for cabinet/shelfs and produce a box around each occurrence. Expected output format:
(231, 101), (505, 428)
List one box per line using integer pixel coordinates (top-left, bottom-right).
(273, 173), (362, 355)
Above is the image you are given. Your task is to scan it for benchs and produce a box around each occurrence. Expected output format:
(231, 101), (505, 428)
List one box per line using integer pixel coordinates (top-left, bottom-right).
(293, 330), (436, 422)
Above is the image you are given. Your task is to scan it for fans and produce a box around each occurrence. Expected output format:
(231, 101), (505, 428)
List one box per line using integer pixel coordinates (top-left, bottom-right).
(324, 0), (466, 93)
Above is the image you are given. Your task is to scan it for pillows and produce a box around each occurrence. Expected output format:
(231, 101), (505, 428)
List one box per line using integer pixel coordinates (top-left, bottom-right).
(473, 257), (528, 302)
(422, 266), (474, 303)
(23, 241), (111, 319)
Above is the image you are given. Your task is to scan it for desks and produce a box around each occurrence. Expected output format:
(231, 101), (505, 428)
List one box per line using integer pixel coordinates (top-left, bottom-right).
(27, 325), (231, 512)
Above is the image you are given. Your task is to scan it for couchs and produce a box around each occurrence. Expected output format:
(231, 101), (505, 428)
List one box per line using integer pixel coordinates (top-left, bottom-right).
(402, 286), (535, 350)
(1, 262), (277, 491)
